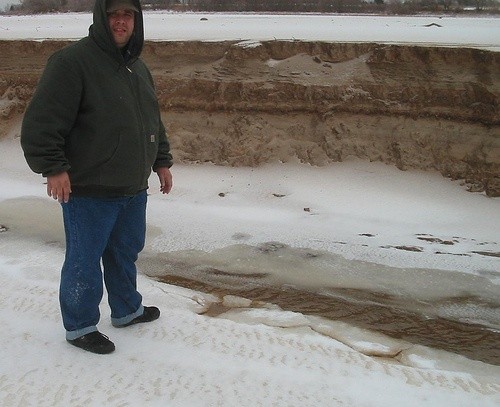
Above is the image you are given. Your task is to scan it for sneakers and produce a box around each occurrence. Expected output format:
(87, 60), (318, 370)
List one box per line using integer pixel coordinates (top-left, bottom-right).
(115, 306), (160, 328)
(66, 331), (115, 354)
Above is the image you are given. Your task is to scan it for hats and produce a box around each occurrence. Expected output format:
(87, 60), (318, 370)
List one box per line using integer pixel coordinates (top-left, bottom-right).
(104, 0), (139, 13)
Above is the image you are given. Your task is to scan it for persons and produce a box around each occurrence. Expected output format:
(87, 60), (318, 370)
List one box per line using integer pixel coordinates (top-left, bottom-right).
(21, 0), (174, 354)
